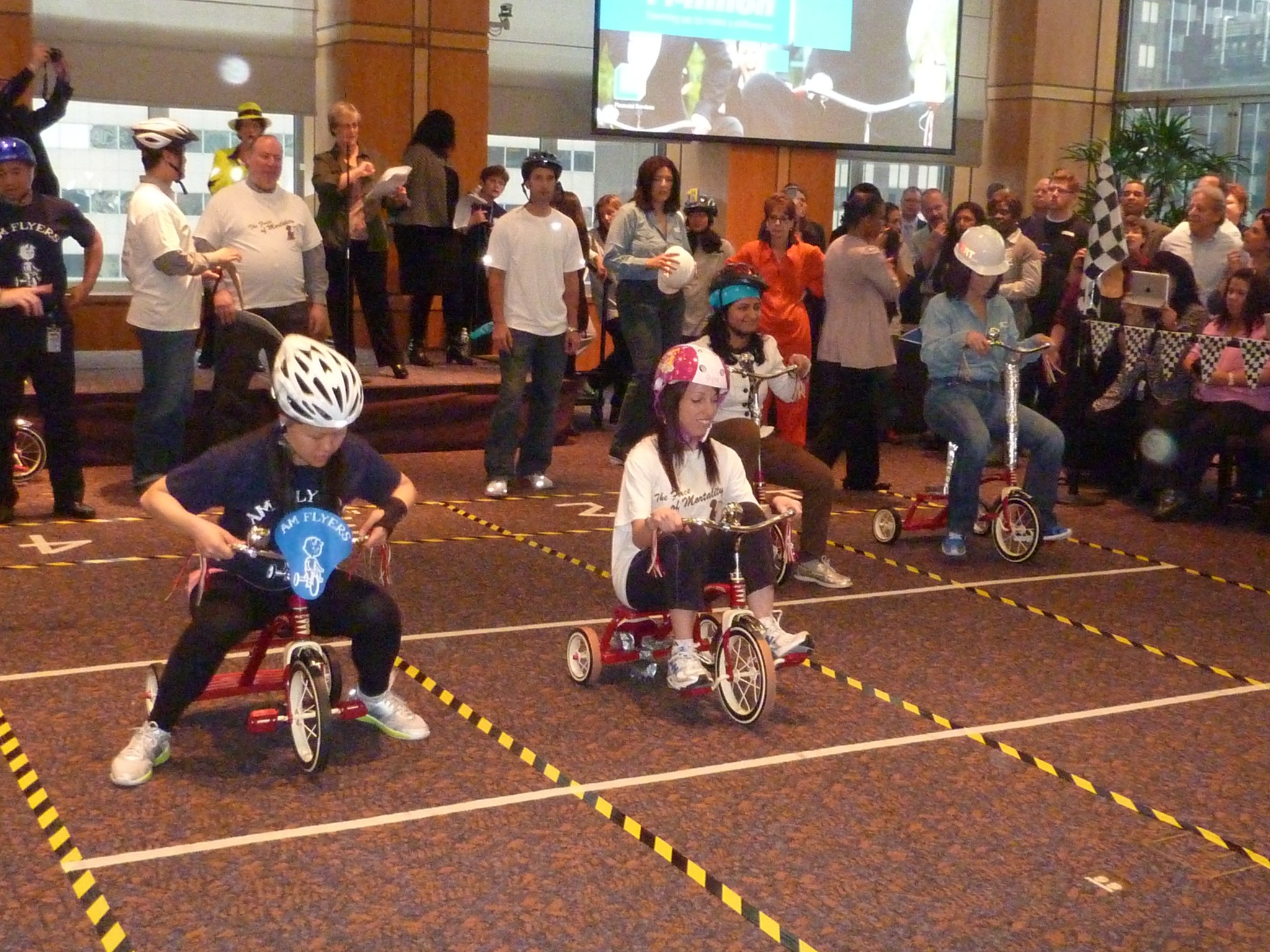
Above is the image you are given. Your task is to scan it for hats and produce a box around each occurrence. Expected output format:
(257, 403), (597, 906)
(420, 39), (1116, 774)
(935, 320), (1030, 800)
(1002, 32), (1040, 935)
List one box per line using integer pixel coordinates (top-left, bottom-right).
(227, 102), (271, 132)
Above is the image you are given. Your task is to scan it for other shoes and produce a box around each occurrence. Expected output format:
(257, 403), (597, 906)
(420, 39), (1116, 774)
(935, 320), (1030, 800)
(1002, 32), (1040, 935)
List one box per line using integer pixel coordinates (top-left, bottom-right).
(1056, 445), (1264, 522)
(0, 506), (15, 524)
(609, 451), (628, 466)
(885, 428), (902, 444)
(525, 473), (554, 489)
(486, 479), (508, 496)
(196, 340), (476, 379)
(53, 501), (95, 519)
(842, 476), (892, 491)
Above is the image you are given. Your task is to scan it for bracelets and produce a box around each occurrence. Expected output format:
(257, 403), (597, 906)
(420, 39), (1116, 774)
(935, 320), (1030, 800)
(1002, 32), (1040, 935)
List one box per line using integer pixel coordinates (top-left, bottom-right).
(568, 326), (578, 332)
(29, 63), (37, 71)
(1228, 373), (1234, 386)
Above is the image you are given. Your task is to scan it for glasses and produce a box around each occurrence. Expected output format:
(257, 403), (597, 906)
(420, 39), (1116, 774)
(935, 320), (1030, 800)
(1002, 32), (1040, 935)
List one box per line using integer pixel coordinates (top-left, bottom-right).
(767, 213), (791, 225)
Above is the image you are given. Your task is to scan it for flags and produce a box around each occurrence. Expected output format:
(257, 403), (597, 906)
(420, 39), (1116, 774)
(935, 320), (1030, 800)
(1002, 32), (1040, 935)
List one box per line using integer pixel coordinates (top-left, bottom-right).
(1083, 146), (1129, 280)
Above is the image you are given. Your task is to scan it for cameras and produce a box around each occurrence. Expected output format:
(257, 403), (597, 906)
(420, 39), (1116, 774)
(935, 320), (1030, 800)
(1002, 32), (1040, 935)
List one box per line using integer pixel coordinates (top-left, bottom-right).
(47, 48), (63, 62)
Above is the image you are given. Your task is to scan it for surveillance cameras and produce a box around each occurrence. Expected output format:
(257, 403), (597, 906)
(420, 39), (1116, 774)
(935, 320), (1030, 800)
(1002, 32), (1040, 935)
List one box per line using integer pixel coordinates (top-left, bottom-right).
(500, 3), (513, 11)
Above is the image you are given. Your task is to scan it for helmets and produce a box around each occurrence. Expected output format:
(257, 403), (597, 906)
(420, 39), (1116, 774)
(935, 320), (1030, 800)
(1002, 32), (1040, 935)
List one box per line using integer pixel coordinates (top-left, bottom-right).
(953, 224), (1010, 276)
(708, 263), (769, 296)
(520, 151), (563, 180)
(683, 194), (718, 216)
(652, 343), (729, 406)
(271, 332), (365, 428)
(0, 137), (37, 163)
(657, 245), (696, 295)
(130, 117), (200, 151)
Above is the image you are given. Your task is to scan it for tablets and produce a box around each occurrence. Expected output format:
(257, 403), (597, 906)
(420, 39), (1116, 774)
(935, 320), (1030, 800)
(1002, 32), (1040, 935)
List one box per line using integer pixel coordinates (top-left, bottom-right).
(1129, 270), (1170, 308)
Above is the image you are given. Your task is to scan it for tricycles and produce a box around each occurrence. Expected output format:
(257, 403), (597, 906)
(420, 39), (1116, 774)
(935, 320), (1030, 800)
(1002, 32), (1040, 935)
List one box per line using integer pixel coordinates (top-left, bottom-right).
(870, 326), (1053, 564)
(12, 418), (48, 482)
(726, 352), (799, 588)
(566, 503), (796, 729)
(146, 523), (372, 777)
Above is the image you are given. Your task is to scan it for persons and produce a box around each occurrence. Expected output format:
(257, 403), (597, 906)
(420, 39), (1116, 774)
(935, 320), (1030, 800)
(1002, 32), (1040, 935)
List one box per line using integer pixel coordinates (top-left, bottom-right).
(603, 152), (693, 466)
(0, 137), (103, 523)
(482, 149), (587, 496)
(110, 334), (430, 784)
(598, 0), (955, 157)
(193, 135), (329, 446)
(919, 225), (1073, 556)
(312, 100), (409, 379)
(123, 116), (244, 498)
(550, 167), (1270, 531)
(684, 263), (854, 588)
(209, 102), (271, 195)
(611, 344), (816, 692)
(0, 41), (74, 293)
(722, 191), (827, 449)
(388, 108), (476, 366)
(458, 165), (509, 358)
(815, 192), (901, 492)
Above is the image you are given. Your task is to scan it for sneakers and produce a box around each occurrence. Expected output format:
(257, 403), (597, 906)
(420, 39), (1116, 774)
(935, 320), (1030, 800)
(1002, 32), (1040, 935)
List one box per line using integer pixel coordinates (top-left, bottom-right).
(941, 532), (966, 556)
(794, 555), (853, 589)
(666, 643), (714, 694)
(758, 610), (815, 659)
(111, 720), (173, 786)
(347, 671), (430, 741)
(1040, 524), (1072, 541)
